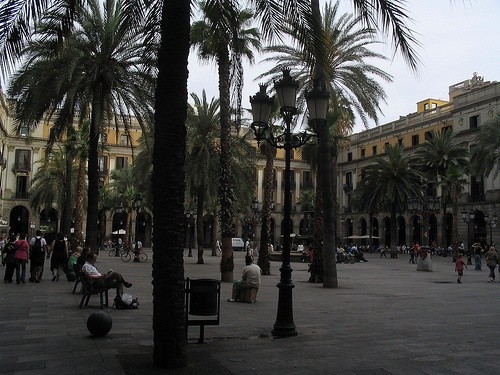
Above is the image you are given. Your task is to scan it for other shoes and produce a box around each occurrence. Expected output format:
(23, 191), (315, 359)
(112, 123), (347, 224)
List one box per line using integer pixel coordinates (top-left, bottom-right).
(122, 281), (132, 288)
(35, 280), (40, 284)
(488, 276), (494, 283)
(51, 277), (56, 281)
(29, 277), (34, 283)
(228, 298), (239, 302)
(457, 279), (461, 284)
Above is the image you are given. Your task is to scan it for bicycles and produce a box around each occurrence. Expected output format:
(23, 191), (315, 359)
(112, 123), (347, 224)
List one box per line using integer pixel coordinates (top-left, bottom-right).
(108, 243), (125, 257)
(121, 246), (148, 263)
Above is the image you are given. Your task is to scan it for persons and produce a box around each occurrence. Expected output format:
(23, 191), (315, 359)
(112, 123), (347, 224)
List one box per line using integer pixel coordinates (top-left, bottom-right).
(227, 255), (262, 302)
(334, 240), (500, 283)
(216, 238), (314, 266)
(0, 230), (142, 299)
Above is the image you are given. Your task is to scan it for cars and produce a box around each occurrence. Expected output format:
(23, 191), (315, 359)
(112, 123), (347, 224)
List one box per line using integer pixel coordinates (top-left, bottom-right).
(232, 238), (244, 251)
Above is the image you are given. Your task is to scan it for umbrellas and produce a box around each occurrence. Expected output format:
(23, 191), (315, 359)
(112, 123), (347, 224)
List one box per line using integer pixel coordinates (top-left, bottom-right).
(111, 229), (126, 234)
(343, 235), (380, 239)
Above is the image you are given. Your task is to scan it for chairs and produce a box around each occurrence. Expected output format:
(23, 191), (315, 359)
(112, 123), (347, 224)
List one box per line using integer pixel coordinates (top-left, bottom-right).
(71, 263), (108, 309)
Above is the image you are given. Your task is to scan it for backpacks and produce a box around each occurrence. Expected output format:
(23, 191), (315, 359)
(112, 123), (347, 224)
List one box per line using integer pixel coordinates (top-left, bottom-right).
(30, 237), (44, 258)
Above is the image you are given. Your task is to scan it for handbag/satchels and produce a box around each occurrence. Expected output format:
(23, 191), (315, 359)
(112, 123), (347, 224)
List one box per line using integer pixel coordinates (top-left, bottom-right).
(114, 294), (140, 309)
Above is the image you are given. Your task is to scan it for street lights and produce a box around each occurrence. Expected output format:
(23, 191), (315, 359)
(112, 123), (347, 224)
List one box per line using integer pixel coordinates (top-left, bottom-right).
(484, 212), (498, 247)
(417, 218), (424, 246)
(248, 67), (331, 337)
(116, 206), (122, 243)
(341, 215), (355, 248)
(461, 208), (475, 265)
(133, 197), (144, 262)
(186, 210), (197, 257)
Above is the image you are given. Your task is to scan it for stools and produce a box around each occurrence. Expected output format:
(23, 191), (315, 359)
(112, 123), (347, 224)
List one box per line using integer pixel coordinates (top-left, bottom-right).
(238, 286), (258, 304)
